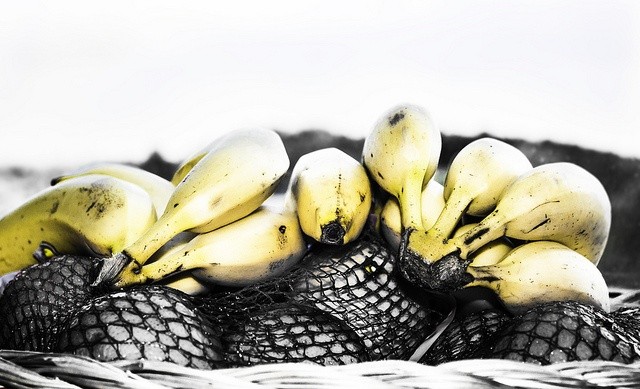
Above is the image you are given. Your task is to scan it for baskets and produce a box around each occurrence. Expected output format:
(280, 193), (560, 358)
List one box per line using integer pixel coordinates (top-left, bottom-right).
(0, 272), (640, 388)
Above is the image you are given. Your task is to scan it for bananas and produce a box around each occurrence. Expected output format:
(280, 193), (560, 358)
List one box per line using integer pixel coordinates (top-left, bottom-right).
(361, 104), (611, 314)
(288, 147), (371, 249)
(0, 129), (305, 291)
